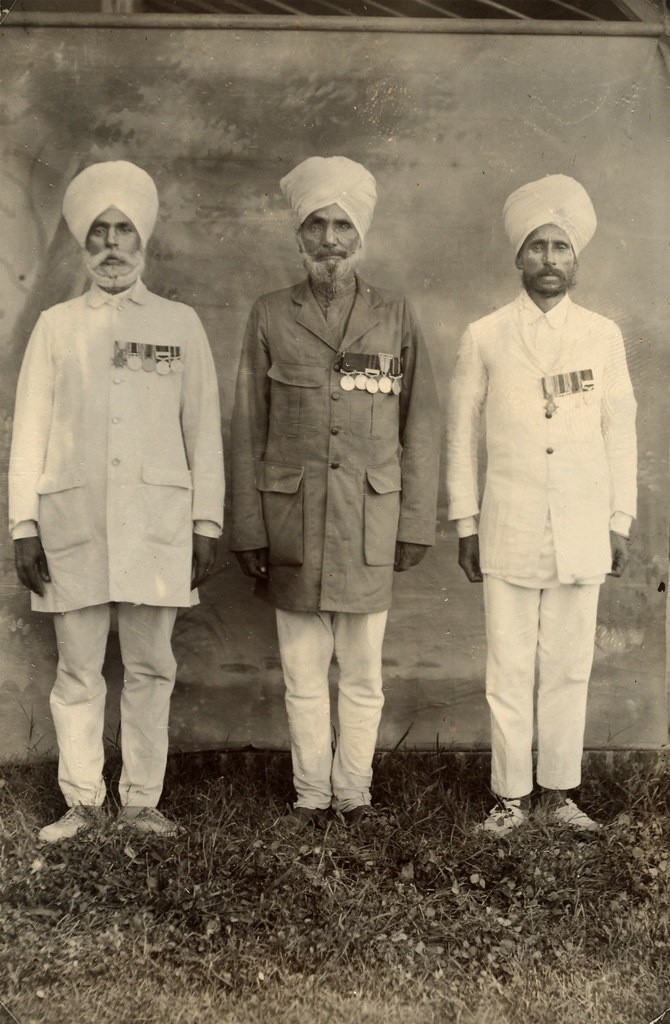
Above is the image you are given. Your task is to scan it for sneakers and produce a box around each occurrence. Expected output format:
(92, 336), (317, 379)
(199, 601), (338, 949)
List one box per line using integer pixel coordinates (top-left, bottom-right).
(535, 797), (603, 837)
(117, 806), (187, 839)
(474, 799), (530, 842)
(38, 805), (106, 843)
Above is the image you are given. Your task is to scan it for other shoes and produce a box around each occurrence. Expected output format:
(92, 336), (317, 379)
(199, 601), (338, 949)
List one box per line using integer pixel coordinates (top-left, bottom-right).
(332, 805), (386, 840)
(272, 806), (330, 837)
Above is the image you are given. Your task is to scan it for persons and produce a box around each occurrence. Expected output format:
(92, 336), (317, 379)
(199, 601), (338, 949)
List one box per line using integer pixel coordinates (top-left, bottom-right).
(231, 155), (443, 852)
(444, 174), (640, 840)
(6, 161), (227, 845)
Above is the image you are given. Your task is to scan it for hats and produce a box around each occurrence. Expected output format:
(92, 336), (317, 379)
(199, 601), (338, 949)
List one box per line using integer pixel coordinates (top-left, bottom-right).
(502, 173), (597, 259)
(61, 160), (159, 251)
(279, 155), (377, 248)
